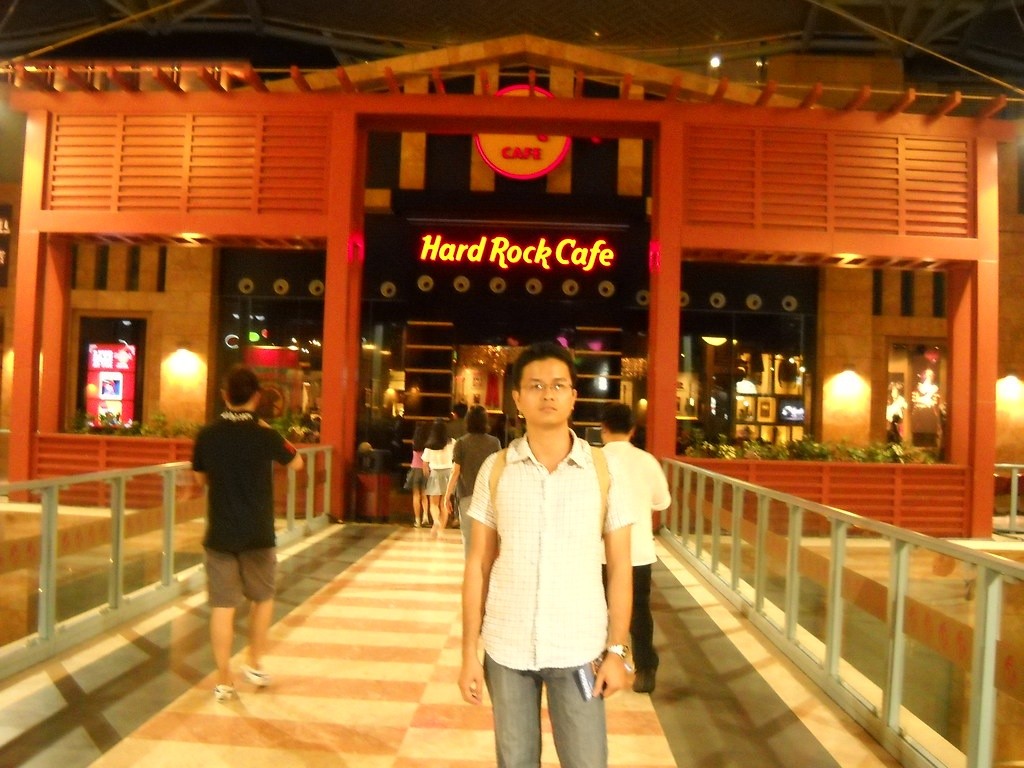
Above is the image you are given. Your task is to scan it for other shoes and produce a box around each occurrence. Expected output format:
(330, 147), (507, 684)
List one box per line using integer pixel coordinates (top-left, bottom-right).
(629, 666), (656, 694)
(243, 664), (272, 687)
(422, 519), (430, 525)
(213, 681), (236, 703)
(451, 520), (461, 529)
(414, 521), (420, 527)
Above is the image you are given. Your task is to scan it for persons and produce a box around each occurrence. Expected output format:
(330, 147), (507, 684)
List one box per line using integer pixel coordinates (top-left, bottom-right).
(888, 414), (903, 443)
(404, 401), (503, 559)
(458, 342), (640, 768)
(192, 366), (305, 703)
(599, 401), (673, 692)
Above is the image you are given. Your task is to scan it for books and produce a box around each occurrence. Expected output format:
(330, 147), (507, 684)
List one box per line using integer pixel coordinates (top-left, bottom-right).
(578, 651), (635, 701)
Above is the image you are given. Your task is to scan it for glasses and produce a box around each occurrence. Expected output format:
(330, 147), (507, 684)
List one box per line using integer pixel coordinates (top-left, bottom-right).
(527, 380), (574, 394)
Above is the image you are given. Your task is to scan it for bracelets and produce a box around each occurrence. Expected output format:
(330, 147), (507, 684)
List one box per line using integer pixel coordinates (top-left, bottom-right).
(606, 644), (630, 659)
